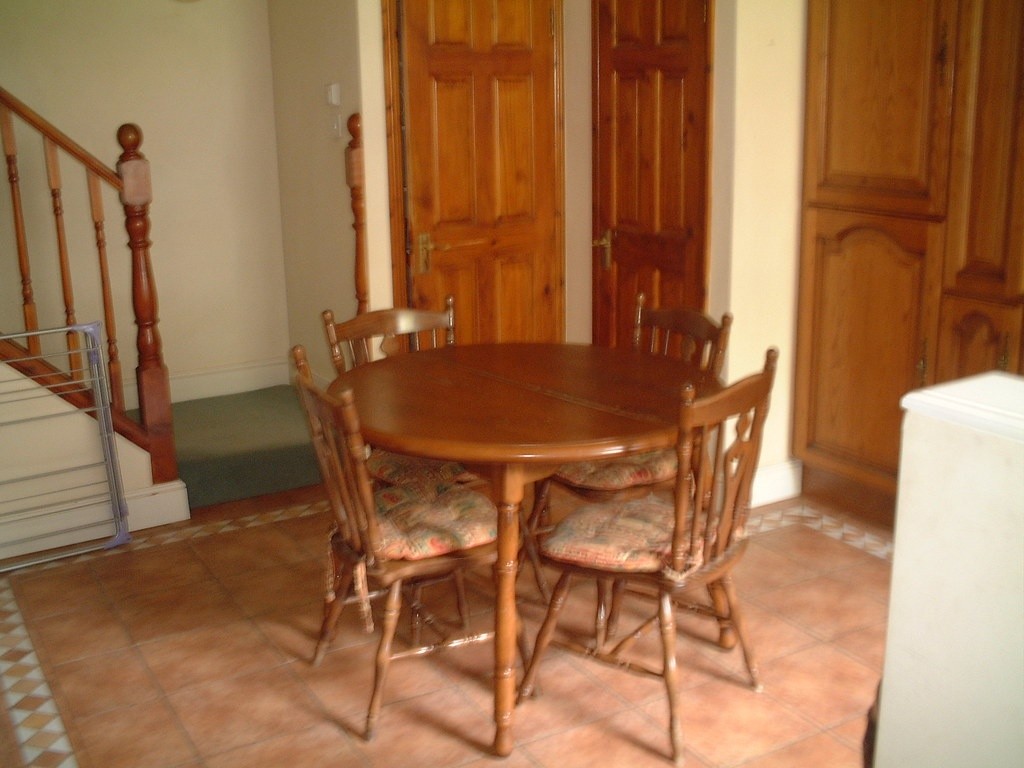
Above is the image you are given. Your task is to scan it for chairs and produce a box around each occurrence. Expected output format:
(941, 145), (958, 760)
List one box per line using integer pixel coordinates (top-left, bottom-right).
(516, 294), (733, 640)
(321, 294), (551, 650)
(516, 348), (779, 767)
(291, 343), (545, 739)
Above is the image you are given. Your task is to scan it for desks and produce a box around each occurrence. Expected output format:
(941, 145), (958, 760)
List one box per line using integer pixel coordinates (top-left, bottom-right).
(328, 342), (726, 757)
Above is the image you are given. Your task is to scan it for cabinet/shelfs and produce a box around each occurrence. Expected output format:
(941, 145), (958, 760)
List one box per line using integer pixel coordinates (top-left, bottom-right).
(790, 0), (1024, 534)
